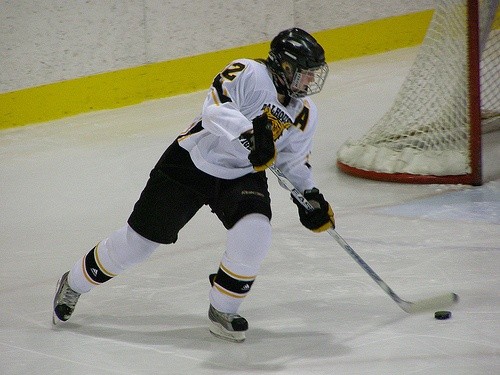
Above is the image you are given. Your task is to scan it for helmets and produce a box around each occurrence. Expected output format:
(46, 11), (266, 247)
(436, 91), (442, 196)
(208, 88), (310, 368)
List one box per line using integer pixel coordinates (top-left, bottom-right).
(267, 26), (328, 107)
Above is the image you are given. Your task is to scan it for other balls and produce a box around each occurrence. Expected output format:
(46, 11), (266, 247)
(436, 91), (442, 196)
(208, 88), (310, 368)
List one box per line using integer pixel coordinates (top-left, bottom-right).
(435, 310), (452, 320)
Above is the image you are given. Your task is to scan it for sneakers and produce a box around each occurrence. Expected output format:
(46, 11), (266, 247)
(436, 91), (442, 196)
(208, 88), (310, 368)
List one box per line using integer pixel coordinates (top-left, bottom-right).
(52, 269), (82, 326)
(206, 304), (249, 343)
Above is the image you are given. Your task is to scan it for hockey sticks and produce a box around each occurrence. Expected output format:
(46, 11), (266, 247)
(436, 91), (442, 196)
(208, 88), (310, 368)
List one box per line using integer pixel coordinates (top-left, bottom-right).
(238, 133), (460, 313)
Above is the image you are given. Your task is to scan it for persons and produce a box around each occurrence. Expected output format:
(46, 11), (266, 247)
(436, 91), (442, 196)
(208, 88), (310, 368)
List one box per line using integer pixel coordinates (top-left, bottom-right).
(54, 28), (336, 344)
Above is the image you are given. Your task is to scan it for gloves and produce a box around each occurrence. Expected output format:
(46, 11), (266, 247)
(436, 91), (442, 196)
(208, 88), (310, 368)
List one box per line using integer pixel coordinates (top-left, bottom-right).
(291, 185), (336, 235)
(245, 114), (279, 172)
